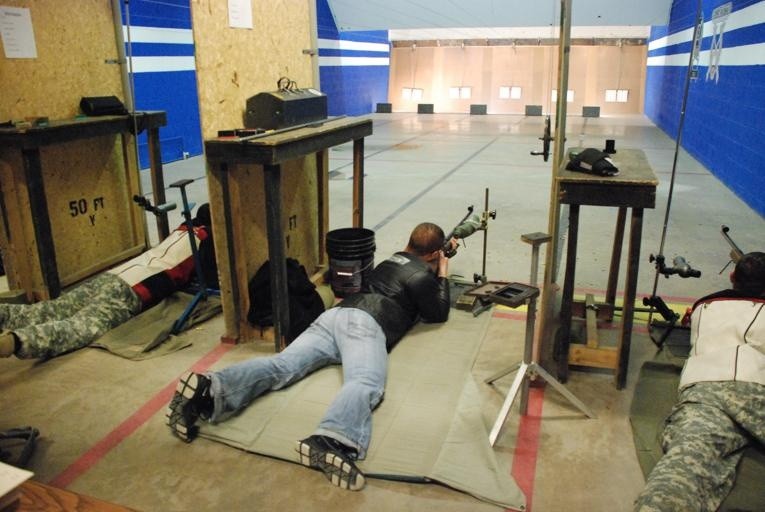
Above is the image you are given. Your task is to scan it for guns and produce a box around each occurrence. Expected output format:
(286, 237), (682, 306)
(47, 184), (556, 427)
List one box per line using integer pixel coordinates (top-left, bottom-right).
(443, 205), (475, 258)
(718, 224), (744, 277)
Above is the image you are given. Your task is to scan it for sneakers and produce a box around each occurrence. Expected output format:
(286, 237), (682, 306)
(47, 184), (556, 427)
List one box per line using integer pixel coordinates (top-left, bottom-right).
(165, 372), (213, 443)
(293, 435), (367, 492)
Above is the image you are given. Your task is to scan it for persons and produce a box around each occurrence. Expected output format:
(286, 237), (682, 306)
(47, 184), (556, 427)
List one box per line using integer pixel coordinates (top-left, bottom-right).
(631, 251), (765, 512)
(163, 222), (458, 492)
(0, 202), (219, 360)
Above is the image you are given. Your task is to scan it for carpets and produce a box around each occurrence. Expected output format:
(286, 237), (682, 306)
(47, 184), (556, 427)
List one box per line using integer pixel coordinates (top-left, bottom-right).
(1, 276), (765, 512)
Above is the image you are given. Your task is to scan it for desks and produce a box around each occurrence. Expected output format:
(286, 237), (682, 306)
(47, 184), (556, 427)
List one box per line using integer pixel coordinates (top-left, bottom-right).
(1, 110), (173, 305)
(204, 111), (377, 353)
(556, 143), (659, 393)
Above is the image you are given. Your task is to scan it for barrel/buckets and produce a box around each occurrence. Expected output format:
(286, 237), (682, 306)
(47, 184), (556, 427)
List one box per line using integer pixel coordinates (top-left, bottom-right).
(326, 228), (375, 299)
